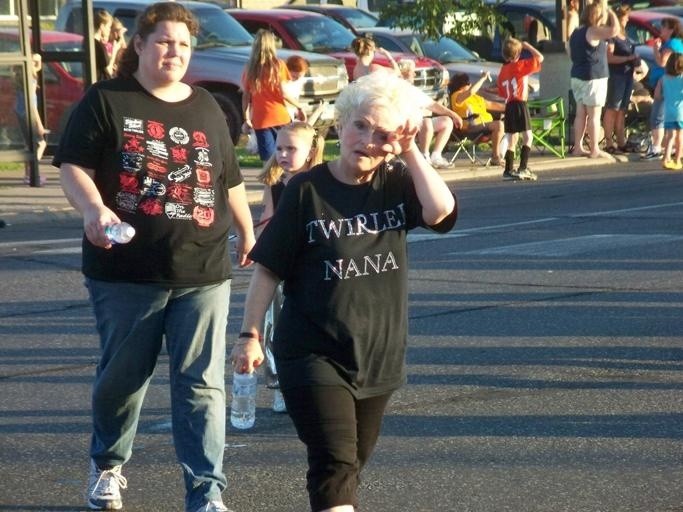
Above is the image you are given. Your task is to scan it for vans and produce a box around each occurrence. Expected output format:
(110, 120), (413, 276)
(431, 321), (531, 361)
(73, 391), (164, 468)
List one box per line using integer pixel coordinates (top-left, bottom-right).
(457, 2), (577, 125)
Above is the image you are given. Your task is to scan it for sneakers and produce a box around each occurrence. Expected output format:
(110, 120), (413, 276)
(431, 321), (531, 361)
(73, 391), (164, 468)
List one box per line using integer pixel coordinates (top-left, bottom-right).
(273, 388), (288, 413)
(196, 499), (234, 512)
(87, 457), (129, 511)
(663, 159), (683, 170)
(502, 167), (537, 181)
(431, 157), (456, 169)
(639, 152), (663, 161)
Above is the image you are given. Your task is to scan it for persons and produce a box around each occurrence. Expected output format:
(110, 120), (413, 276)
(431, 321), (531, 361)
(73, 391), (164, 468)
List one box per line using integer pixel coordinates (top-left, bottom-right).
(50, 1), (256, 511)
(241, 27), (308, 172)
(256, 122), (325, 414)
(11, 54), (48, 187)
(80, 8), (121, 93)
(226, 67), (462, 511)
(278, 52), (309, 124)
(101, 17), (128, 78)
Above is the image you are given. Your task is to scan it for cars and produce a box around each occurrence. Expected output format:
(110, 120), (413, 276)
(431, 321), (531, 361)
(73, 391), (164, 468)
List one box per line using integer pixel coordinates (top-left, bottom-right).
(619, 10), (683, 45)
(643, 5), (683, 20)
(273, 4), (383, 30)
(355, 27), (542, 102)
(0, 29), (105, 135)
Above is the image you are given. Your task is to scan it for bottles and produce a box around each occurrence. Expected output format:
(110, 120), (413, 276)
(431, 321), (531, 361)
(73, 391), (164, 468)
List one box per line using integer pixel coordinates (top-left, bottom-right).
(479, 70), (492, 84)
(104, 220), (136, 248)
(230, 364), (258, 430)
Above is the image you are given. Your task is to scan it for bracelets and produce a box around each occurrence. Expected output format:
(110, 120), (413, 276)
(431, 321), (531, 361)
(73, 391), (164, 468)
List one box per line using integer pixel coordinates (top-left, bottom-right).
(297, 106), (302, 111)
(238, 329), (262, 341)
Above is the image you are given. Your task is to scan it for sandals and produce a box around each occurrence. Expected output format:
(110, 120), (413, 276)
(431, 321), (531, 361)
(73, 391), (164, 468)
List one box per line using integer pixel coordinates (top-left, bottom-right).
(606, 146), (623, 155)
(618, 144), (637, 152)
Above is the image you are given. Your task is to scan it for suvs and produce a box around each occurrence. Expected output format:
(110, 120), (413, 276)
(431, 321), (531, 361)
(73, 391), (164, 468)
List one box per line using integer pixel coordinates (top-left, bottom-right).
(53, 0), (349, 149)
(223, 6), (451, 104)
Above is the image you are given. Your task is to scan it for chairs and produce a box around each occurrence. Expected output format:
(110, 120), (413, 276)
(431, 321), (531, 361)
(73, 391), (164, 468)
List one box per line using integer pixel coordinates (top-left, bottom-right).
(448, 94), (650, 171)
